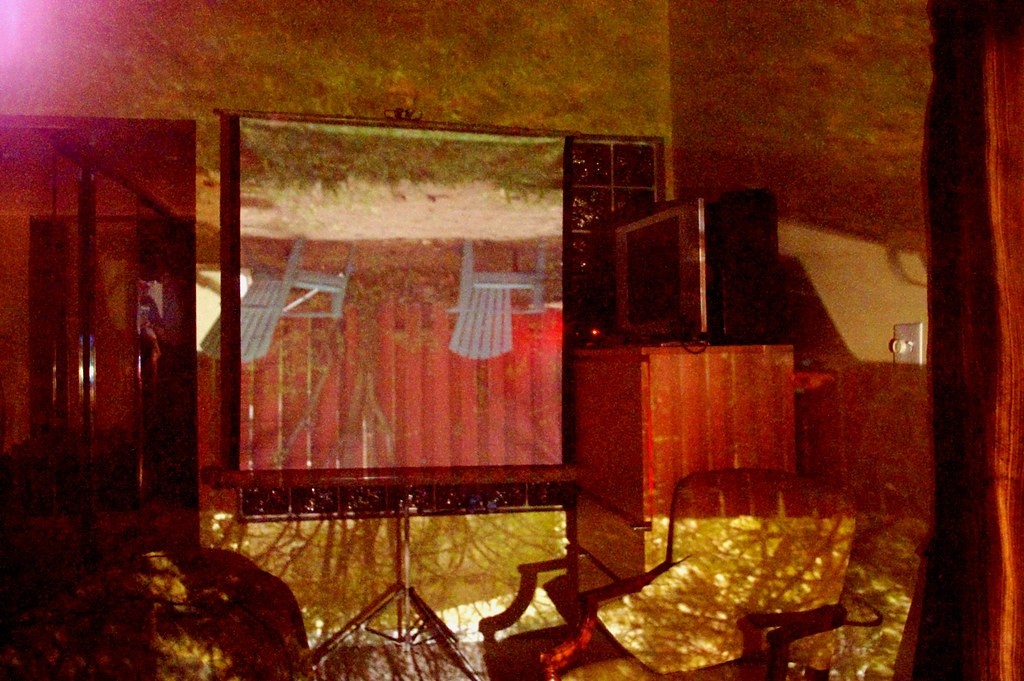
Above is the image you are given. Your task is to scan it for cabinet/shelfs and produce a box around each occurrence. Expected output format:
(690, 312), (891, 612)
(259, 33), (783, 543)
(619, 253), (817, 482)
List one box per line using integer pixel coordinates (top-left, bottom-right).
(574, 344), (797, 591)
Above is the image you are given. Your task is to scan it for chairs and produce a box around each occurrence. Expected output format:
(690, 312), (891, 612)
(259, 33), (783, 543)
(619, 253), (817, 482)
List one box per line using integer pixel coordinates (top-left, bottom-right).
(424, 239), (547, 358)
(198, 231), (360, 362)
(539, 464), (857, 681)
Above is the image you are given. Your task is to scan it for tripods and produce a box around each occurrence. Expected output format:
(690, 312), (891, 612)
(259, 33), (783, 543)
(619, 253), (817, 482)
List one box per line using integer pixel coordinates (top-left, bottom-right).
(303, 518), (481, 681)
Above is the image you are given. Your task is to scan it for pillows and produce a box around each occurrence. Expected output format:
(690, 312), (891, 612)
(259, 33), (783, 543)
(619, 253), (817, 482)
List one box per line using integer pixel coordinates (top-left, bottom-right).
(597, 551), (777, 671)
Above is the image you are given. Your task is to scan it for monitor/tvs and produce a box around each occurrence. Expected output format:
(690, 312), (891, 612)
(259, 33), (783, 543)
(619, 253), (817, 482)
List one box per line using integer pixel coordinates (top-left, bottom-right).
(609, 192), (716, 345)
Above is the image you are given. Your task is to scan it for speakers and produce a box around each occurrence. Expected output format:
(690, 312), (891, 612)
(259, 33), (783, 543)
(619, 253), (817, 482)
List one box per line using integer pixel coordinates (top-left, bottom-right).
(712, 185), (779, 343)
